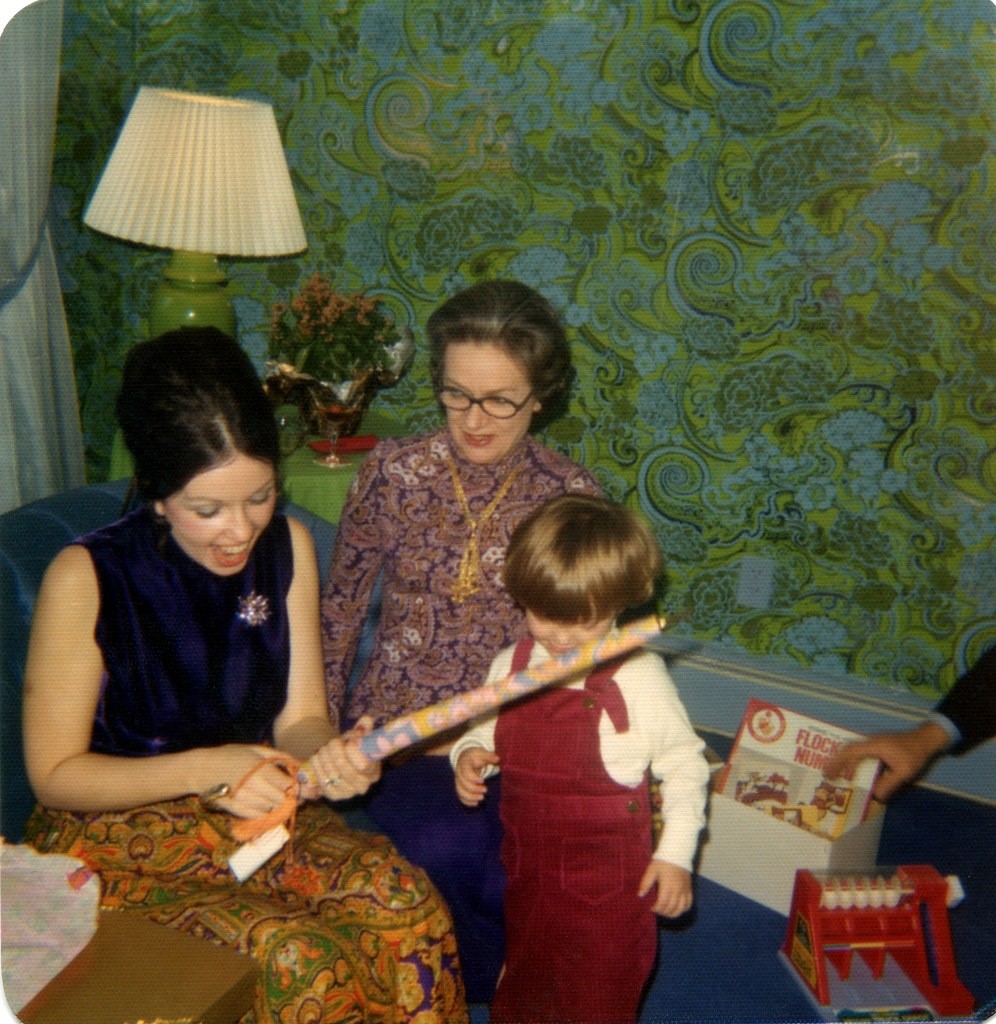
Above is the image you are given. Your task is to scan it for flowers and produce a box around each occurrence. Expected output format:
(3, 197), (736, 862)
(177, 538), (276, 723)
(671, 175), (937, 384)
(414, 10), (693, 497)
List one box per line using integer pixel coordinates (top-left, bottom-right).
(261, 270), (400, 388)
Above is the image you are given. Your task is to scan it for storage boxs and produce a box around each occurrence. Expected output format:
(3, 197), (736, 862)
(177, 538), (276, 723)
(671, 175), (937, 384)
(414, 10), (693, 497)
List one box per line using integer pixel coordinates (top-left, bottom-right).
(692, 763), (885, 920)
(18, 906), (259, 1024)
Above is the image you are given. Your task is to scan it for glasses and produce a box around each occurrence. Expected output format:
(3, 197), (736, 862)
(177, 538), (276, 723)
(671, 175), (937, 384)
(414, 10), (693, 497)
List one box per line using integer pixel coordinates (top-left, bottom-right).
(432, 376), (538, 420)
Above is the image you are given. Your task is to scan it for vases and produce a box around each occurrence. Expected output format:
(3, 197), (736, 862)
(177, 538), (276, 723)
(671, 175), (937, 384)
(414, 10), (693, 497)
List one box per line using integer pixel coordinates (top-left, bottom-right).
(307, 389), (365, 468)
(264, 345), (415, 440)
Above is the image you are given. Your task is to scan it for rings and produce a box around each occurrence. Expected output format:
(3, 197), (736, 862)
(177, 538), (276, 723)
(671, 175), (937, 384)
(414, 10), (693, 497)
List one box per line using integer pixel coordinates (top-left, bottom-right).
(337, 774), (343, 782)
(265, 802), (275, 815)
(324, 778), (334, 785)
(284, 782), (294, 793)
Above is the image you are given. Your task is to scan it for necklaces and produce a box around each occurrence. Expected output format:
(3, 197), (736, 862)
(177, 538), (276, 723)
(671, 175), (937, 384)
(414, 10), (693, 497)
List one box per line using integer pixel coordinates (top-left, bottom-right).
(442, 443), (521, 601)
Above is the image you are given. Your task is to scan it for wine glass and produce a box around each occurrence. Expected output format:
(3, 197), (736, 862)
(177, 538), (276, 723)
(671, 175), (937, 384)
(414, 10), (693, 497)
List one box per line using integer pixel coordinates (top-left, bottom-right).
(312, 389), (363, 465)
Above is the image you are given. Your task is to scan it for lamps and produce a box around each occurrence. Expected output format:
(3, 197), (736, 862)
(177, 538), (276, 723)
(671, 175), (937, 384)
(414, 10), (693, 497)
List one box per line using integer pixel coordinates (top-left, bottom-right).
(82, 87), (309, 340)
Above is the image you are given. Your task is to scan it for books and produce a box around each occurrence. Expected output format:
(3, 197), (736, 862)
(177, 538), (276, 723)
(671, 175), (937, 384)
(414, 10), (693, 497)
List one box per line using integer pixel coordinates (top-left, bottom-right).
(712, 695), (882, 843)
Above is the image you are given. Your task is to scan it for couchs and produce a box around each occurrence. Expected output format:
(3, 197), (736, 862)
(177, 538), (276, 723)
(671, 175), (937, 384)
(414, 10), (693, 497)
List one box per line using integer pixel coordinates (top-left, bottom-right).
(0, 478), (385, 844)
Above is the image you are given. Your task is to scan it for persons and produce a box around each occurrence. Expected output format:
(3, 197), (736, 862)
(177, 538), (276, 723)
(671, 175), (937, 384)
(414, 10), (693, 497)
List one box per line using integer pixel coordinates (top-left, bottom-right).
(449, 492), (711, 1024)
(319, 281), (606, 991)
(21, 325), (469, 1024)
(825, 643), (996, 801)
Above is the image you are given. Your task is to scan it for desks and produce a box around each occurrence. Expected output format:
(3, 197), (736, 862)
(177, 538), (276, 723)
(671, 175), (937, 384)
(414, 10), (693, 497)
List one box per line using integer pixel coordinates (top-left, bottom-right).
(114, 402), (409, 524)
